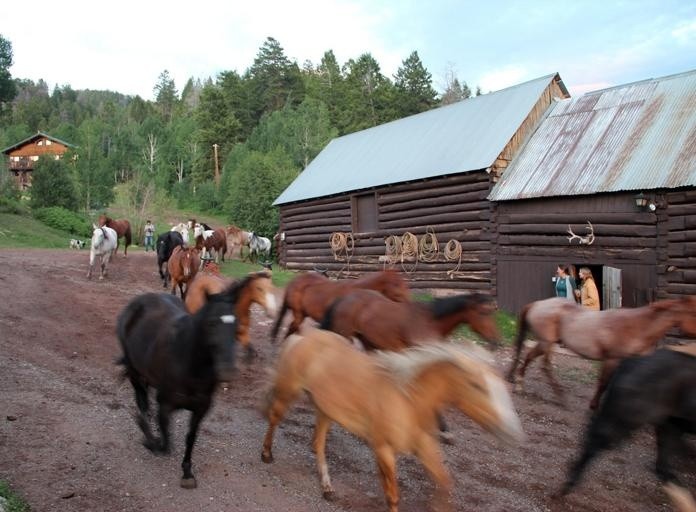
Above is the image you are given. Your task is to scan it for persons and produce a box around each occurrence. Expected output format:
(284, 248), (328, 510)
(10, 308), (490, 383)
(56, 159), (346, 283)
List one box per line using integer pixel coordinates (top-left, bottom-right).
(550, 264), (577, 302)
(143, 219), (156, 252)
(573, 267), (601, 311)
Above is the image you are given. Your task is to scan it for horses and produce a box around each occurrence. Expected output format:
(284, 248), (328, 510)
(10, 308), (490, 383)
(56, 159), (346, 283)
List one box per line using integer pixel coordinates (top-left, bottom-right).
(319, 288), (500, 445)
(506, 293), (696, 410)
(269, 270), (412, 341)
(184, 263), (280, 350)
(98, 211), (130, 258)
(86, 223), (117, 281)
(258, 325), (524, 512)
(557, 343), (696, 511)
(115, 279), (241, 490)
(155, 218), (274, 301)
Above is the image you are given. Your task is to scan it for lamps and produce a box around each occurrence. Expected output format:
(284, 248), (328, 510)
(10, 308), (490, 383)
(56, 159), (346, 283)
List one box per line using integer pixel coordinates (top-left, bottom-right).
(635, 191), (650, 211)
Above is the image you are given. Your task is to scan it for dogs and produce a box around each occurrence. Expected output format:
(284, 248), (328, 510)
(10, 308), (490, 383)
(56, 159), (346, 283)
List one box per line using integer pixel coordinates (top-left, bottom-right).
(69, 238), (85, 251)
